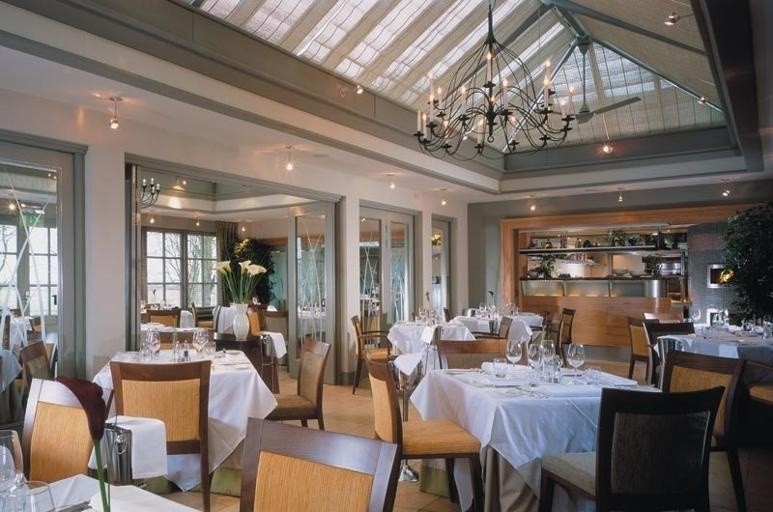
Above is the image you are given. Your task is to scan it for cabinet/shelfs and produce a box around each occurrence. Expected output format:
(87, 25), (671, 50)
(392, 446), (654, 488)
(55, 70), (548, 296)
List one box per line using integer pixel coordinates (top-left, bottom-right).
(512, 231), (656, 297)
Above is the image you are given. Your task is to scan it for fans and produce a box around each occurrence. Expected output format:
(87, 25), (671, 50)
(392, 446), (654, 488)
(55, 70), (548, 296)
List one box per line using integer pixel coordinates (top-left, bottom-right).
(532, 35), (642, 126)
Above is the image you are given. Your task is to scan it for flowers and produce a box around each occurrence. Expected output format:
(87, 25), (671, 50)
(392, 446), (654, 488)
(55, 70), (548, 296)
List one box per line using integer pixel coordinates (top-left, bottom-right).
(211, 260), (267, 304)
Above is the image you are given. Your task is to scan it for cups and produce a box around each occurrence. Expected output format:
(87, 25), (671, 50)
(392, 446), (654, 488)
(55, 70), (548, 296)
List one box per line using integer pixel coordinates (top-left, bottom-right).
(418, 306), (428, 323)
(567, 343), (585, 389)
(2, 479), (54, 512)
(204, 341), (216, 354)
(139, 326), (161, 362)
(689, 307), (702, 325)
(0, 430), (23, 511)
(538, 340), (555, 379)
(479, 301), (496, 320)
(527, 343), (545, 381)
(191, 328), (209, 359)
(493, 358), (507, 379)
(507, 338), (523, 379)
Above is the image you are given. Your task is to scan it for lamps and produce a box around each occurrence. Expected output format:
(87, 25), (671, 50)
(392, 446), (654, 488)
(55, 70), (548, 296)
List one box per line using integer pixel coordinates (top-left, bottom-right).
(140, 177), (160, 209)
(339, 83), (363, 97)
(664, 12), (694, 26)
(108, 96), (122, 130)
(697, 94), (712, 105)
(410, 2), (576, 162)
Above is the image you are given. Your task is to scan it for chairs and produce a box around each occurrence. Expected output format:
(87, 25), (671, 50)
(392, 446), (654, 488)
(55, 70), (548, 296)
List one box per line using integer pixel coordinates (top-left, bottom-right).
(20, 377), (115, 490)
(263, 336), (332, 430)
(109, 360), (211, 512)
(28, 316), (41, 332)
(641, 320), (696, 388)
(261, 309), (289, 372)
(213, 339), (263, 380)
(25, 329), (42, 346)
(625, 315), (659, 384)
(351, 315), (392, 395)
(1, 314), (10, 351)
(560, 307), (576, 344)
(43, 340), (57, 380)
(239, 416), (403, 511)
(19, 340), (53, 394)
(146, 308), (182, 328)
(429, 339), (529, 369)
(661, 349), (749, 512)
(364, 359), (484, 511)
(537, 384), (727, 512)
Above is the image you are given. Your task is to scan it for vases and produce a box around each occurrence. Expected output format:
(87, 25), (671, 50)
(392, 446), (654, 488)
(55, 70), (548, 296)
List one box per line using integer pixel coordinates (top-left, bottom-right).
(232, 304), (249, 341)
